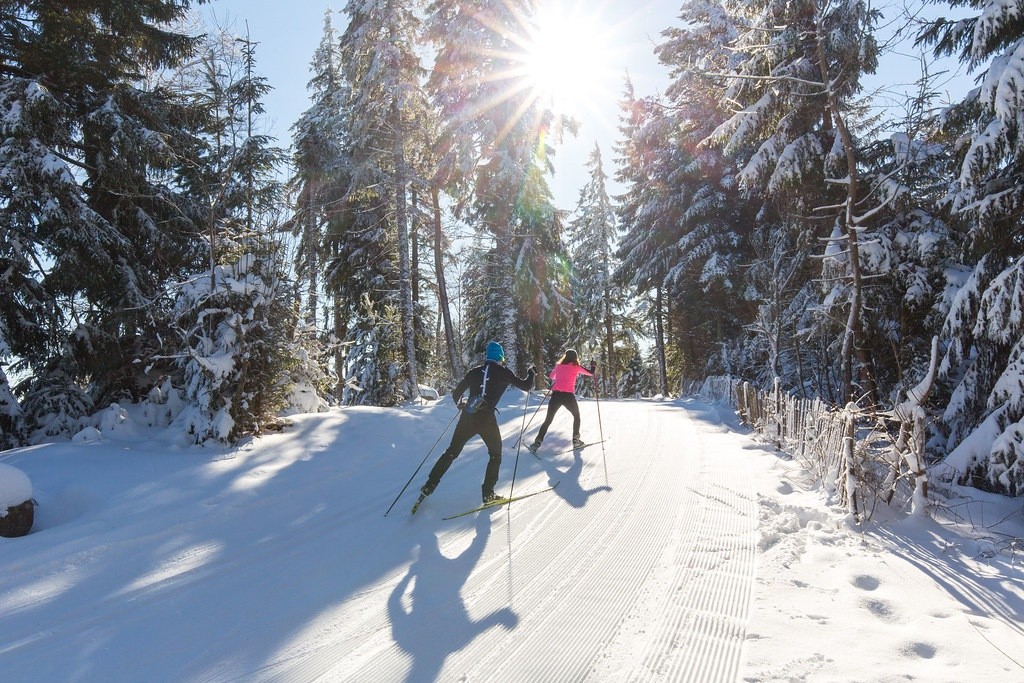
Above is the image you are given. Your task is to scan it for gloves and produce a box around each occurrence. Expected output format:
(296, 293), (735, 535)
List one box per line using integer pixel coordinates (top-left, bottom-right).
(527, 366), (537, 376)
(591, 361), (597, 370)
(456, 400), (467, 410)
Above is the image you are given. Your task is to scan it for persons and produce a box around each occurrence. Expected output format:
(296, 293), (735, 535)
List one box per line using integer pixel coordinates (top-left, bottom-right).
(529, 349), (597, 456)
(417, 339), (535, 505)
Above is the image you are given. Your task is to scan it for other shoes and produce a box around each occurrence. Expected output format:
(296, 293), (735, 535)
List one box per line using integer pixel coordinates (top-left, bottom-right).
(483, 492), (504, 505)
(573, 439), (583, 447)
(530, 441), (539, 452)
(421, 482), (434, 497)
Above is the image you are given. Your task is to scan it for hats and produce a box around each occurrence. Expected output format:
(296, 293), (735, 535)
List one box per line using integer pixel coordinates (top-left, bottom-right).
(487, 341), (504, 362)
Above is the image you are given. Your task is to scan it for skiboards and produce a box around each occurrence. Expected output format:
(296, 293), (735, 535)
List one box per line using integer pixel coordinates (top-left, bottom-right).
(410, 479), (563, 521)
(522, 436), (607, 460)
